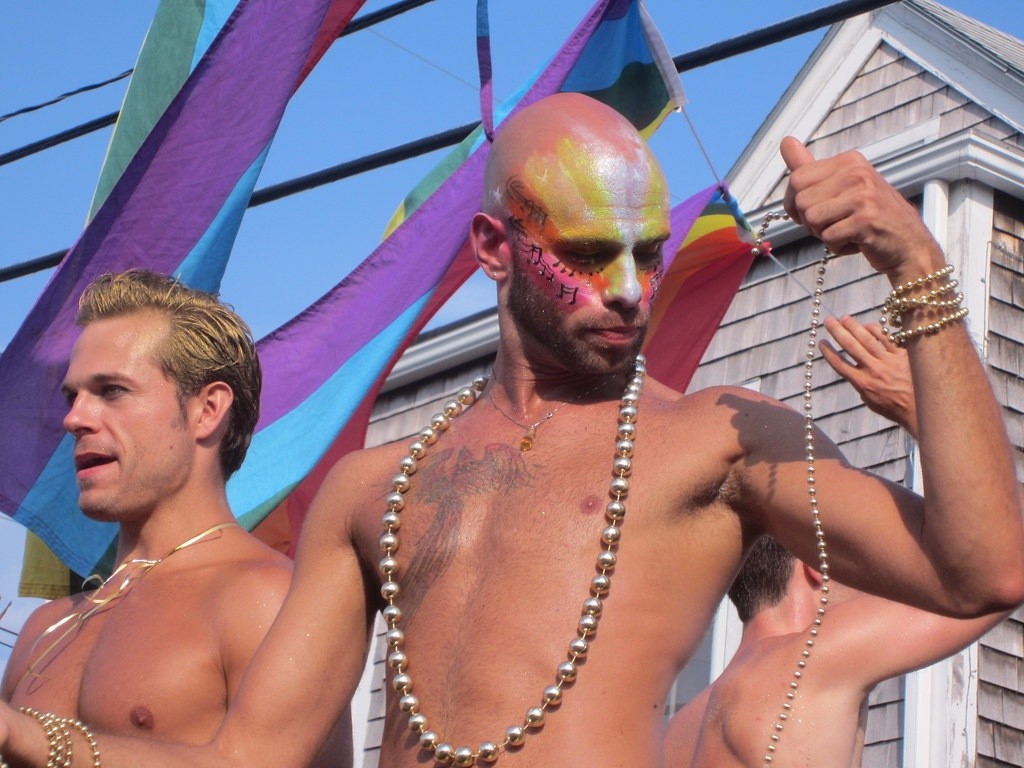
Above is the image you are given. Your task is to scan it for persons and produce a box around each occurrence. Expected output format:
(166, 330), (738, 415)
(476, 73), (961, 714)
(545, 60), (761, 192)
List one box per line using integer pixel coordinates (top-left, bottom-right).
(661, 533), (1023, 767)
(0, 270), (352, 768)
(0, 91), (1024, 768)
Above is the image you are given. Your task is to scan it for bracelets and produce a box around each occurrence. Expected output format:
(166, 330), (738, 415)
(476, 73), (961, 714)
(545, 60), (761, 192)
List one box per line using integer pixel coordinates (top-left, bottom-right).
(879, 266), (968, 346)
(19, 707), (101, 768)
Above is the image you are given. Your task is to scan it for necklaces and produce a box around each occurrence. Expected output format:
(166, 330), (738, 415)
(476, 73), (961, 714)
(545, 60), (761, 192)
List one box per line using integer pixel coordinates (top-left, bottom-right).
(489, 367), (617, 452)
(13, 523), (239, 697)
(380, 354), (646, 768)
